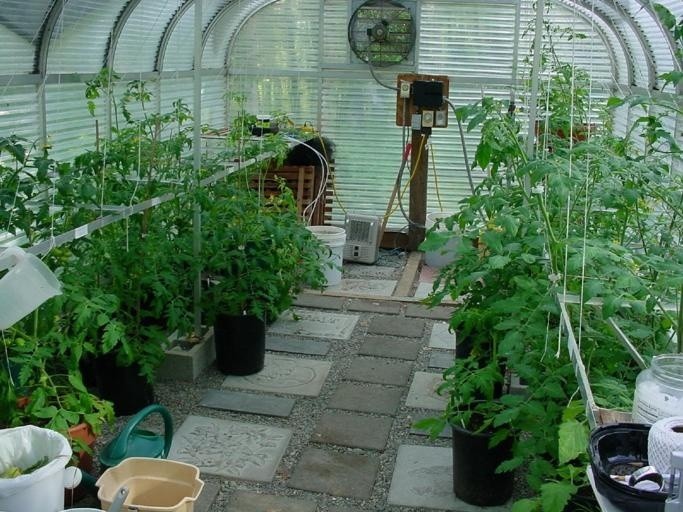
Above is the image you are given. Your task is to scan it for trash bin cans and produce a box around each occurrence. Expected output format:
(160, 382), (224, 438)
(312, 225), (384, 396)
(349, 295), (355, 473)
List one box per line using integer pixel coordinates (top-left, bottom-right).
(0, 425), (72, 512)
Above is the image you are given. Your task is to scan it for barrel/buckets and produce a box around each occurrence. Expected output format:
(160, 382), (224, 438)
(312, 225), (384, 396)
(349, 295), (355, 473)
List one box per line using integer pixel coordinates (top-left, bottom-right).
(632, 354), (683, 425)
(96, 459), (203, 511)
(305, 225), (346, 286)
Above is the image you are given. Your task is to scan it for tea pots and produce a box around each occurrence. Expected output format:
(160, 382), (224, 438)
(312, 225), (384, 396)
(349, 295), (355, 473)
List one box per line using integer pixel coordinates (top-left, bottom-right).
(0, 246), (63, 329)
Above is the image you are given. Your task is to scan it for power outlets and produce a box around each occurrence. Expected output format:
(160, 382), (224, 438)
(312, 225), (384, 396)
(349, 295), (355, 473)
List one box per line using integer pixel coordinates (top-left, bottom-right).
(400, 82), (410, 98)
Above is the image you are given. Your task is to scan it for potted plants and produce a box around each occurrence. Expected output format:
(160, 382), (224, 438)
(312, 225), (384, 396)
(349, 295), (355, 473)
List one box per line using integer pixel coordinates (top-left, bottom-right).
(0, 36), (344, 473)
(411, 0), (683, 512)
